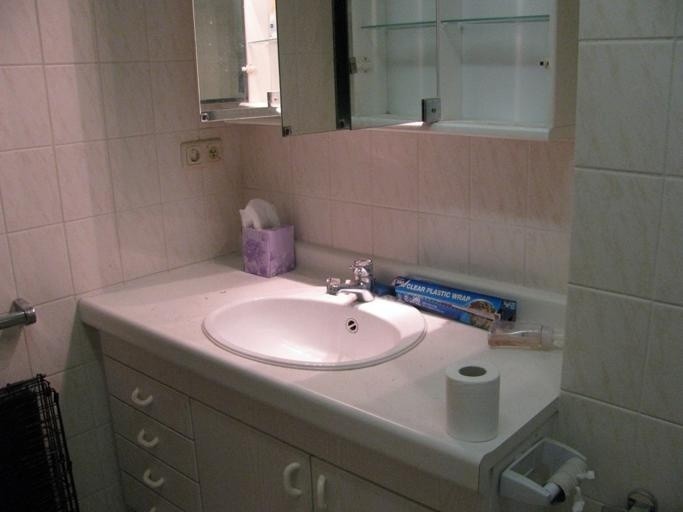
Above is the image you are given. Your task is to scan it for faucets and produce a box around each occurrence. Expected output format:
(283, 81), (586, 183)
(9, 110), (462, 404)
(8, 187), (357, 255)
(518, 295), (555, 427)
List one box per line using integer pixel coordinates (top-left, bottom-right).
(325, 257), (376, 302)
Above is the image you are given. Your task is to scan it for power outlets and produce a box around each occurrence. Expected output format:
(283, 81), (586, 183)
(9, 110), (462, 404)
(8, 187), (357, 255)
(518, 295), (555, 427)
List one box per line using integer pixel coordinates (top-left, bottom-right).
(180, 138), (224, 168)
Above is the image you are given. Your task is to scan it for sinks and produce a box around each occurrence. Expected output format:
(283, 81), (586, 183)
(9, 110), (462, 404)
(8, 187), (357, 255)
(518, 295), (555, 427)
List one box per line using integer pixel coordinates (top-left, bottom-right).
(201, 291), (428, 372)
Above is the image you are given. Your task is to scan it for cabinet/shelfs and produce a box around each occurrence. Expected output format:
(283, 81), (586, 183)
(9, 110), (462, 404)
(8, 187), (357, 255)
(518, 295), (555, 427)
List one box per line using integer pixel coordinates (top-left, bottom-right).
(189, 397), (560, 511)
(79, 256), (203, 512)
